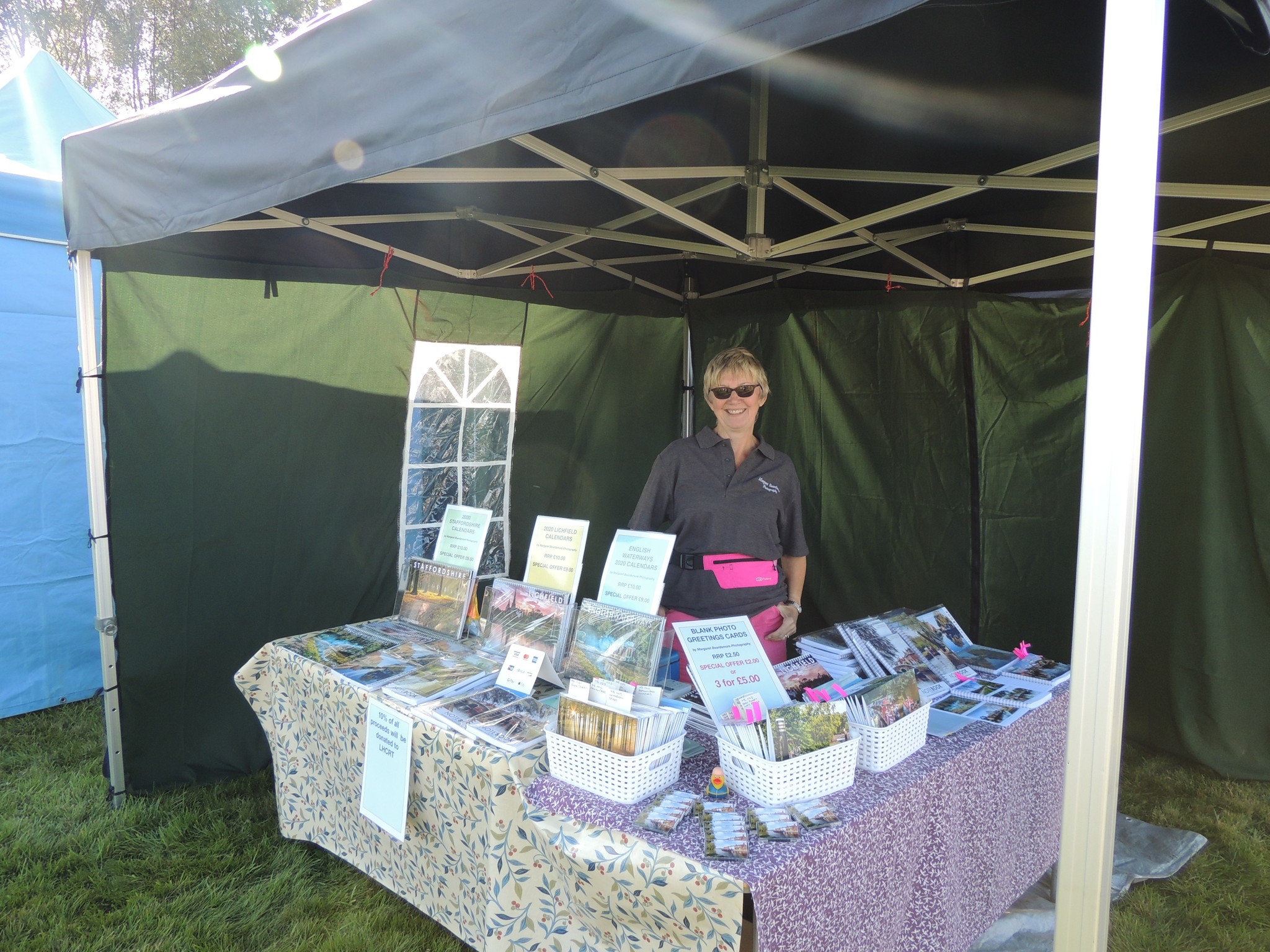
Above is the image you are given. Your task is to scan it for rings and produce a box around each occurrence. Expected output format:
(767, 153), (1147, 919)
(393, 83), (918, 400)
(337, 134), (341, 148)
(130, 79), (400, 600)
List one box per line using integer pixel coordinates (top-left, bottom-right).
(787, 635), (789, 638)
(784, 635), (788, 639)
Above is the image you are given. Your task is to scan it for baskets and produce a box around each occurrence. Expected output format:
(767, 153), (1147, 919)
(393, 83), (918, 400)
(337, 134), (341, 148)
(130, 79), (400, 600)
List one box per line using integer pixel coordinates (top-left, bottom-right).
(847, 692), (933, 774)
(544, 716), (687, 805)
(716, 725), (862, 810)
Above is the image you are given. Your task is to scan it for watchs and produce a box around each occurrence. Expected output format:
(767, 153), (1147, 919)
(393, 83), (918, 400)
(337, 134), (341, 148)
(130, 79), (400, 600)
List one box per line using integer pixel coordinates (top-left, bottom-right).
(786, 600), (802, 615)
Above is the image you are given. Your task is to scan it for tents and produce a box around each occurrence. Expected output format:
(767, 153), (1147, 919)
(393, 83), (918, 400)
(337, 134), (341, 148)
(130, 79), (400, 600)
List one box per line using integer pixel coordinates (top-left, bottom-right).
(0, 45), (118, 718)
(59, 0), (1270, 952)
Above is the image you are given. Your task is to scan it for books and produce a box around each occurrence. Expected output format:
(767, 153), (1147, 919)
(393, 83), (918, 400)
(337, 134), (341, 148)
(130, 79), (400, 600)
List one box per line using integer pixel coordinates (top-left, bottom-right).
(281, 557), (1070, 775)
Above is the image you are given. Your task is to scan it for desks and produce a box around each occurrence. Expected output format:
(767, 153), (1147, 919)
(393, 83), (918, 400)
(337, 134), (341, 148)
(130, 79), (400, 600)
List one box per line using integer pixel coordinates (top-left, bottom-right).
(234, 613), (1070, 952)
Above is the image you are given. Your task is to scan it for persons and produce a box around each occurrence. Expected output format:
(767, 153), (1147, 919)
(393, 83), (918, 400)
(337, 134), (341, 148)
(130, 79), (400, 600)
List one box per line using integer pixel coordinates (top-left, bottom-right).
(628, 347), (809, 688)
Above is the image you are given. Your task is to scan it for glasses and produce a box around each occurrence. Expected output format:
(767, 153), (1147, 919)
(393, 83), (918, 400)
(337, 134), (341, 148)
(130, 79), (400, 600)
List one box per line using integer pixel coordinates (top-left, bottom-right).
(707, 384), (761, 400)
